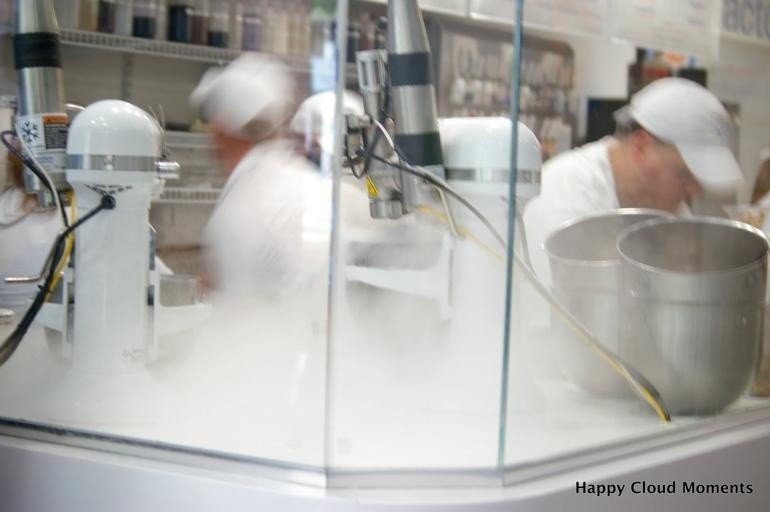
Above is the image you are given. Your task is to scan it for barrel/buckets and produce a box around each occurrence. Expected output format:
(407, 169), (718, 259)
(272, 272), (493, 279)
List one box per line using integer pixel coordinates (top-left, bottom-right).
(541, 206), (677, 396)
(613, 214), (770, 418)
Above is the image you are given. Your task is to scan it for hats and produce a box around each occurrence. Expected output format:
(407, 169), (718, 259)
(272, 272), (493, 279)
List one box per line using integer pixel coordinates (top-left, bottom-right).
(187, 53), (297, 131)
(292, 90), (365, 152)
(628, 75), (744, 195)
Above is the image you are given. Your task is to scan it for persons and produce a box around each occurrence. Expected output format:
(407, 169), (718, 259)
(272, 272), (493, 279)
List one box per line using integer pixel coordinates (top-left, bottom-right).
(1, 138), (73, 318)
(522, 77), (743, 329)
(189, 46), (375, 307)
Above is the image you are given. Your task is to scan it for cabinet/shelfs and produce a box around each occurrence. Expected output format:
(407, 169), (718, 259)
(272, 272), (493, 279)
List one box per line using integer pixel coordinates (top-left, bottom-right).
(0, 0), (577, 240)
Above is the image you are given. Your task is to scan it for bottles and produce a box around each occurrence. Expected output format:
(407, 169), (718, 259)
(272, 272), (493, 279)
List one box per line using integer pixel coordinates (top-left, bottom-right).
(53, 0), (330, 58)
(449, 76), (571, 117)
(347, 13), (388, 62)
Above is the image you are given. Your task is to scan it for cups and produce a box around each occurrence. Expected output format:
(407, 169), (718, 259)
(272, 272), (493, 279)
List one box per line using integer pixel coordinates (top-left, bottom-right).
(540, 208), (769, 417)
